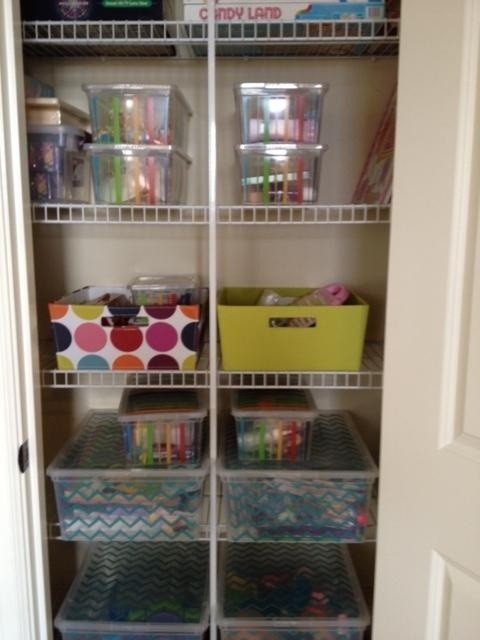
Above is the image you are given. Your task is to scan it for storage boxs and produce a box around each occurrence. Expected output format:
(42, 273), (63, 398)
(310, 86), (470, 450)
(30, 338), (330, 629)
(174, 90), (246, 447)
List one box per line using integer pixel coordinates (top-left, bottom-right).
(233, 142), (331, 208)
(25, 79), (191, 213)
(119, 373), (203, 468)
(48, 286), (208, 372)
(232, 79), (330, 146)
(46, 408), (205, 544)
(217, 285), (370, 372)
(51, 540), (211, 638)
(182, 0), (386, 56)
(20, 0), (177, 58)
(218, 540), (372, 639)
(219, 407), (380, 542)
(228, 386), (319, 466)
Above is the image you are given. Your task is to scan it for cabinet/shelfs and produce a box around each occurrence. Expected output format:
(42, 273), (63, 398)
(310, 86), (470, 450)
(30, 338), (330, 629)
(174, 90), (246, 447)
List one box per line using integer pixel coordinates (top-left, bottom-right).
(10, 0), (403, 638)
